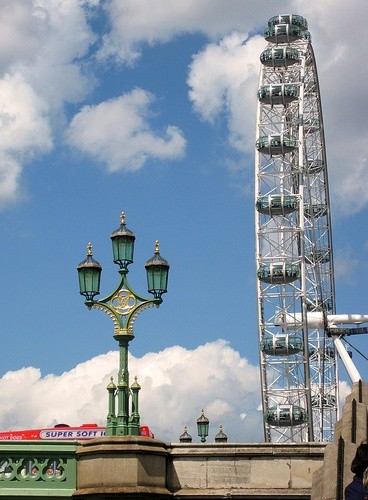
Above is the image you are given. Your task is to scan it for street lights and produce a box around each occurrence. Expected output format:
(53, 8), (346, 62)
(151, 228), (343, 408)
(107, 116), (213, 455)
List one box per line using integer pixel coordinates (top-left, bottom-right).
(77, 211), (171, 438)
